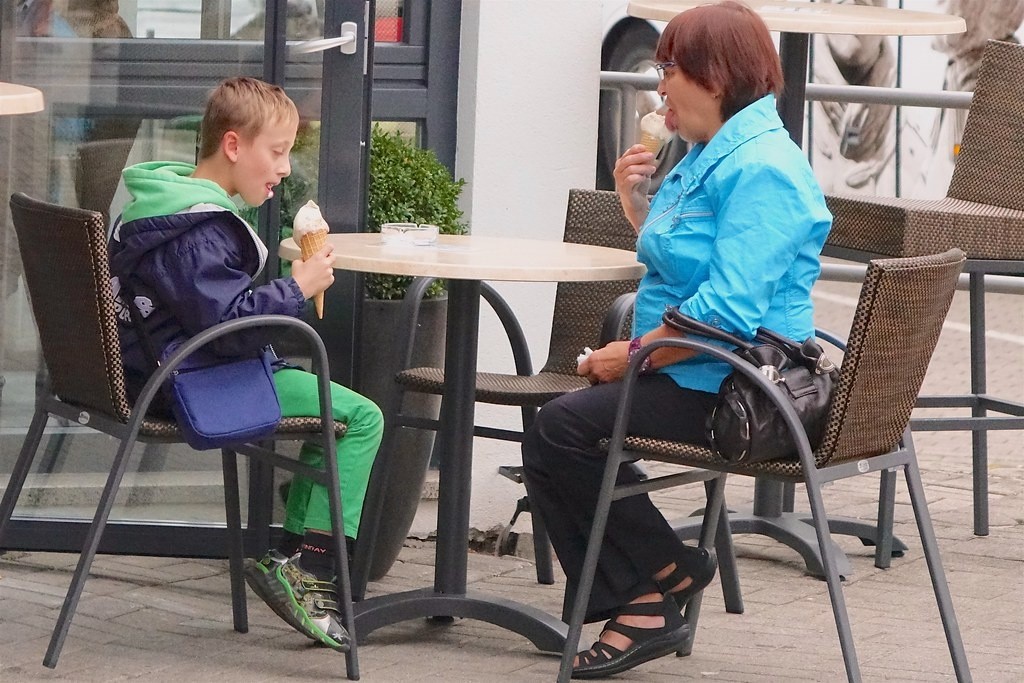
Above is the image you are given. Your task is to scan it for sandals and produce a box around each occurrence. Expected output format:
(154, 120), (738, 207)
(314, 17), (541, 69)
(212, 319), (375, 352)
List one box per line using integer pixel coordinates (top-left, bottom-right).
(655, 546), (714, 607)
(571, 591), (691, 678)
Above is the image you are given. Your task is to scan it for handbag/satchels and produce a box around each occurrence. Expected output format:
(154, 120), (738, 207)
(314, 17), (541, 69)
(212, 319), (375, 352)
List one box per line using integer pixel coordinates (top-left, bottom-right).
(663, 305), (840, 464)
(155, 335), (281, 451)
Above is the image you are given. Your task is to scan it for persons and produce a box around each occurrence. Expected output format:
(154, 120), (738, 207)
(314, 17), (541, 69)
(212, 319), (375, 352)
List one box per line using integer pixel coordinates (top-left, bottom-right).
(103, 74), (385, 651)
(524, 0), (833, 679)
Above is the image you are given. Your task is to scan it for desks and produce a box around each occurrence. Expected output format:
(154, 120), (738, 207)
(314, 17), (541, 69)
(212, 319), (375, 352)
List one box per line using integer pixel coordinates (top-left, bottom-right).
(626, 0), (968, 149)
(275, 229), (650, 656)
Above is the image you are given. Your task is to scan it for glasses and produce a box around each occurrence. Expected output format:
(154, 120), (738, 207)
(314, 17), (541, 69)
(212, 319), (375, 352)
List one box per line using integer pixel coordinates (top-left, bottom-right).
(652, 60), (678, 78)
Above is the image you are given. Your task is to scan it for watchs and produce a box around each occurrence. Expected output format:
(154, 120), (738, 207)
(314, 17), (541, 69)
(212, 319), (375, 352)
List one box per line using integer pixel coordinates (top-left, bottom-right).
(627, 336), (651, 378)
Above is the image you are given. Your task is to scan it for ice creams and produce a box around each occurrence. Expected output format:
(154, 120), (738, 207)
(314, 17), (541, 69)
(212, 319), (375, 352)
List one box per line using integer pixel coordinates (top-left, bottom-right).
(640, 111), (669, 163)
(292, 199), (331, 319)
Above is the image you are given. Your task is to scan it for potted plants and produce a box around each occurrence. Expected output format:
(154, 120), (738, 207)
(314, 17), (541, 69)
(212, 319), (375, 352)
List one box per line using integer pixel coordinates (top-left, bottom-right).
(236, 119), (474, 586)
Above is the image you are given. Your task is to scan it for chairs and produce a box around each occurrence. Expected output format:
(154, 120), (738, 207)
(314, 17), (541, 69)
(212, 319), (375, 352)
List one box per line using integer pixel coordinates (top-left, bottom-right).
(820, 39), (1024, 535)
(348, 188), (655, 604)
(596, 249), (975, 683)
(1, 192), (364, 682)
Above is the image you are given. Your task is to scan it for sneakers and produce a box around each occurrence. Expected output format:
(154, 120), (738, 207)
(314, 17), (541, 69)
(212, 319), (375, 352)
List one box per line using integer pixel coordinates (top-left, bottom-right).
(243, 546), (350, 653)
